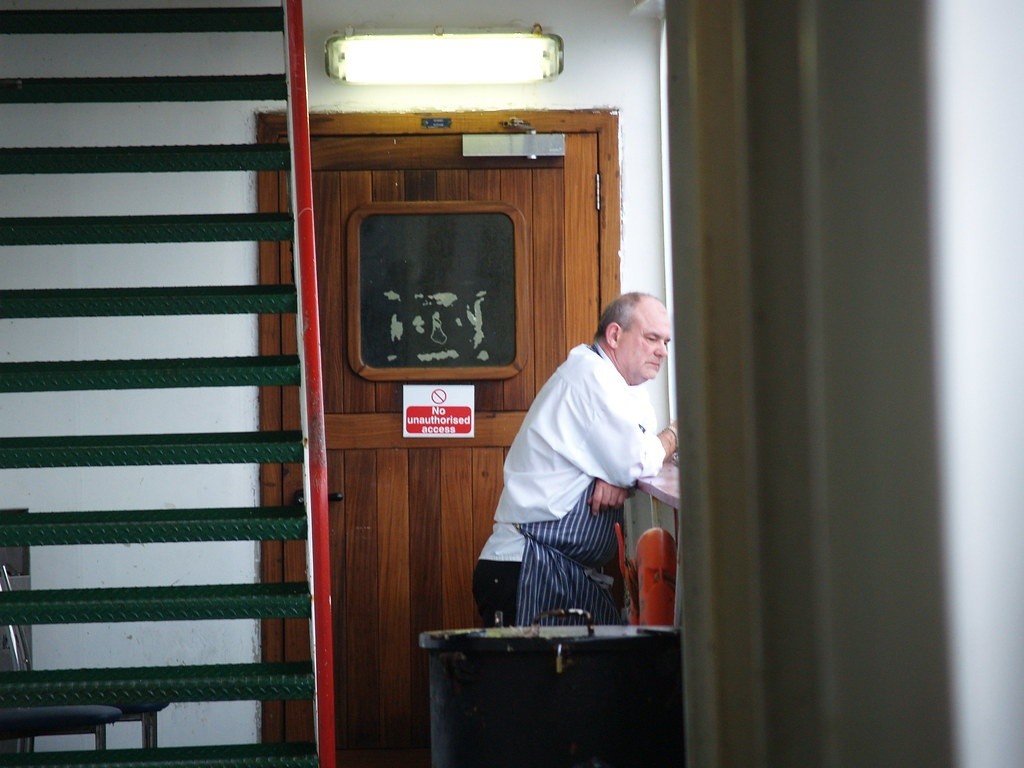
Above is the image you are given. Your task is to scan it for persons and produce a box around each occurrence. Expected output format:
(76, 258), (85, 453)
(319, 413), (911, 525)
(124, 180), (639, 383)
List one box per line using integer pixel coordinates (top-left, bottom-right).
(473, 291), (669, 629)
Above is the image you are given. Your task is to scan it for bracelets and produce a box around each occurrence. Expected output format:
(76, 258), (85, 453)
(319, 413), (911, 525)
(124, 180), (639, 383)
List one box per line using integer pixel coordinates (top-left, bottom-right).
(663, 425), (680, 449)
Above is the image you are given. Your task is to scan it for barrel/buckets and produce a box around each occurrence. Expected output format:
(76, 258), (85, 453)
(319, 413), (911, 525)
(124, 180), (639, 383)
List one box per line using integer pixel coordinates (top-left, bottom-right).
(419, 608), (684, 768)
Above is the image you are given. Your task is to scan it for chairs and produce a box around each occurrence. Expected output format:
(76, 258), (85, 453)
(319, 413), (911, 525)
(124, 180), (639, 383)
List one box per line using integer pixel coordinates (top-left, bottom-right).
(0, 564), (169, 753)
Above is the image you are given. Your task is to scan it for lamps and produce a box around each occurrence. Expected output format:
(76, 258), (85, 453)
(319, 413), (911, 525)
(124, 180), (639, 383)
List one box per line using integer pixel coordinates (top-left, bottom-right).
(324, 23), (565, 85)
